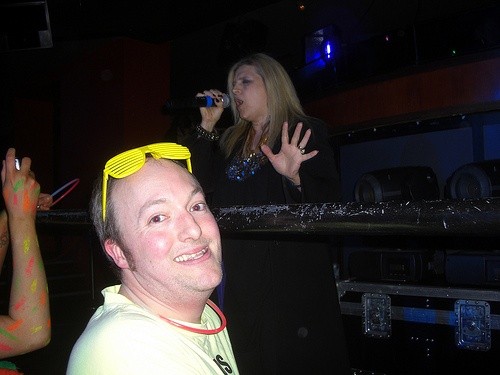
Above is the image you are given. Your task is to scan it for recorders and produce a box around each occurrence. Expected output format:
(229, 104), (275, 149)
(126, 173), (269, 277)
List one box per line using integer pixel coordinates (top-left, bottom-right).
(347, 248), (446, 287)
(446, 251), (500, 291)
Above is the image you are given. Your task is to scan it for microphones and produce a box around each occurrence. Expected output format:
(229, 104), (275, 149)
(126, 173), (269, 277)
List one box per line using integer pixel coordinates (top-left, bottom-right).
(191, 93), (230, 108)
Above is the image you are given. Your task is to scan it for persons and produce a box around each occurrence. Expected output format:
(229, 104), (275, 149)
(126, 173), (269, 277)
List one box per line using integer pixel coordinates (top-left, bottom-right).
(0, 147), (52, 375)
(177, 52), (321, 203)
(65, 153), (239, 375)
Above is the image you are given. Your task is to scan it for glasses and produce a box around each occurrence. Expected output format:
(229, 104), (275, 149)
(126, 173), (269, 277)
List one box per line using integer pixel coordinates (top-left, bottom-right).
(101, 141), (193, 223)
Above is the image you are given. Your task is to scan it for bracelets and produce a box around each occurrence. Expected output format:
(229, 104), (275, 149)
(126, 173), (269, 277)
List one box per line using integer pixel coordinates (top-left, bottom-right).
(194, 124), (221, 142)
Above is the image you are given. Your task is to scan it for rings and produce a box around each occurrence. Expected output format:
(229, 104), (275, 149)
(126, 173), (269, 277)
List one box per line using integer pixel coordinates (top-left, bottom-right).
(36, 202), (42, 211)
(297, 144), (306, 155)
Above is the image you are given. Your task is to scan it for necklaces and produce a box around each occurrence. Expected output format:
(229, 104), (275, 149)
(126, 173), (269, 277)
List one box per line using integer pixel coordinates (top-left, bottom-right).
(245, 126), (269, 158)
